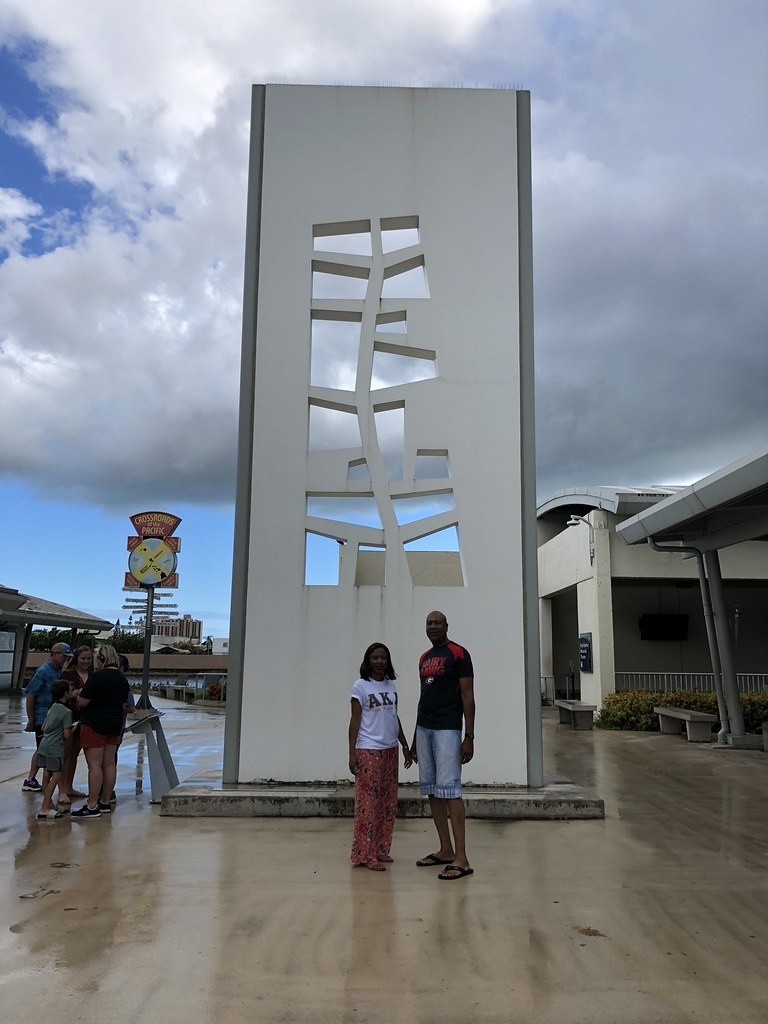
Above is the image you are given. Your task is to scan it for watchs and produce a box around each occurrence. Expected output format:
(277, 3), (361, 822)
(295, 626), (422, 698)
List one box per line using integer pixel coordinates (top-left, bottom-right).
(465, 733), (474, 739)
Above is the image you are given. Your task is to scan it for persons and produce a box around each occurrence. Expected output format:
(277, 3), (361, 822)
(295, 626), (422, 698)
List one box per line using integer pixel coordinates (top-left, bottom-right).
(70, 645), (130, 818)
(37, 680), (69, 818)
(349, 642), (412, 870)
(410, 611), (476, 879)
(87, 655), (134, 803)
(23, 642), (94, 799)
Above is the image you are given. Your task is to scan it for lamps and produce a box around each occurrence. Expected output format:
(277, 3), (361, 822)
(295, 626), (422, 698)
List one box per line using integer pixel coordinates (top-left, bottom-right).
(567, 515), (594, 542)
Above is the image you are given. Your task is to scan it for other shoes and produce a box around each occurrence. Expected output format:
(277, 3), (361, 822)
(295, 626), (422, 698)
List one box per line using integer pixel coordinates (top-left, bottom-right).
(360, 861), (386, 871)
(377, 855), (393, 863)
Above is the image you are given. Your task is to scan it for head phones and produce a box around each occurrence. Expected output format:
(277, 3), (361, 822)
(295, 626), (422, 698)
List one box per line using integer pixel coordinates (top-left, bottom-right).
(99, 647), (106, 663)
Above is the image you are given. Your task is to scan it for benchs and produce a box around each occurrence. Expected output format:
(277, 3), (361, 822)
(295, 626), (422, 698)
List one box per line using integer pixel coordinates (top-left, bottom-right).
(554, 700), (597, 730)
(654, 707), (717, 743)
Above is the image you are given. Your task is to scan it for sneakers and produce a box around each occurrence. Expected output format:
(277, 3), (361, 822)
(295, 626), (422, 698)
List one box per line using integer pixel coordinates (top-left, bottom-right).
(22, 778), (42, 791)
(71, 803), (101, 817)
(98, 800), (111, 812)
(88, 790), (118, 804)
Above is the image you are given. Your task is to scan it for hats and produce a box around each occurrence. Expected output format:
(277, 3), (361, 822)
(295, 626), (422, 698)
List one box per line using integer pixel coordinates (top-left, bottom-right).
(52, 642), (74, 656)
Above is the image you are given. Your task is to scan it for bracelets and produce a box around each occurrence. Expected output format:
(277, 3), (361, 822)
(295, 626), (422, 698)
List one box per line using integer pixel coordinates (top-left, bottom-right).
(402, 746), (408, 749)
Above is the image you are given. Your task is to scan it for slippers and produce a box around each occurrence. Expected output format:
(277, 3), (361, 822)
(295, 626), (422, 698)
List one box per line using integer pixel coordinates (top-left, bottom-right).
(416, 853), (454, 866)
(438, 865), (474, 880)
(36, 808), (63, 819)
(57, 805), (66, 813)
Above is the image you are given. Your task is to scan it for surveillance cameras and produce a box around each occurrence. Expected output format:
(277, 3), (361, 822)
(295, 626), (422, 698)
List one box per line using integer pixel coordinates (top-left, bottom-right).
(567, 519), (580, 526)
